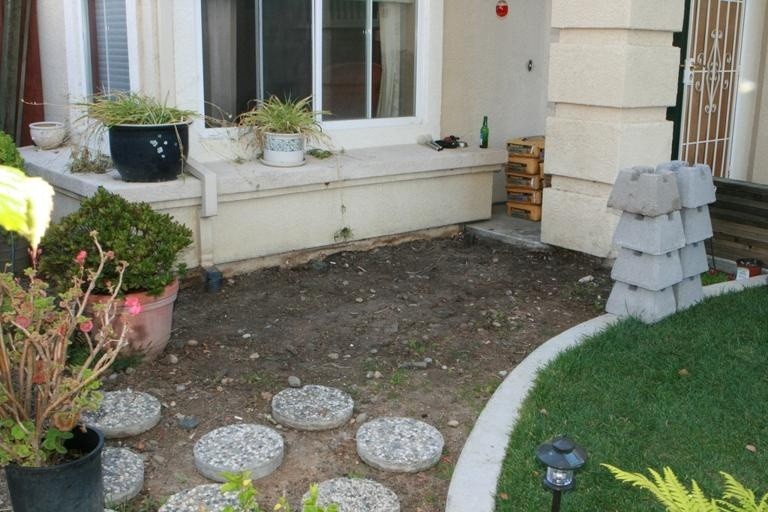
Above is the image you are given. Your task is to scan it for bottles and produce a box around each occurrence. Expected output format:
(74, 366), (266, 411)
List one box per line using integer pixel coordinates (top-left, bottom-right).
(479, 115), (488, 148)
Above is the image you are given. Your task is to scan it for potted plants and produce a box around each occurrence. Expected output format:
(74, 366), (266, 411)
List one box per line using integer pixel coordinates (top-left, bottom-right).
(36, 186), (193, 364)
(0, 130), (30, 284)
(61, 82), (231, 182)
(232, 89), (336, 167)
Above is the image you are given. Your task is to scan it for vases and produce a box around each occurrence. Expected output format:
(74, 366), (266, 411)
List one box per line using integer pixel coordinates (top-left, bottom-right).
(7, 424), (106, 510)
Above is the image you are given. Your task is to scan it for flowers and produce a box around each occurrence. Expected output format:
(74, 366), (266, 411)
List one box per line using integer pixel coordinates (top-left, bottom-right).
(0, 230), (135, 466)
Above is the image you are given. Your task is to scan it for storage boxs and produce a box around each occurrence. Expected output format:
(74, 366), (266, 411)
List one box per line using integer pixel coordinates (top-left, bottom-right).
(506, 136), (551, 221)
